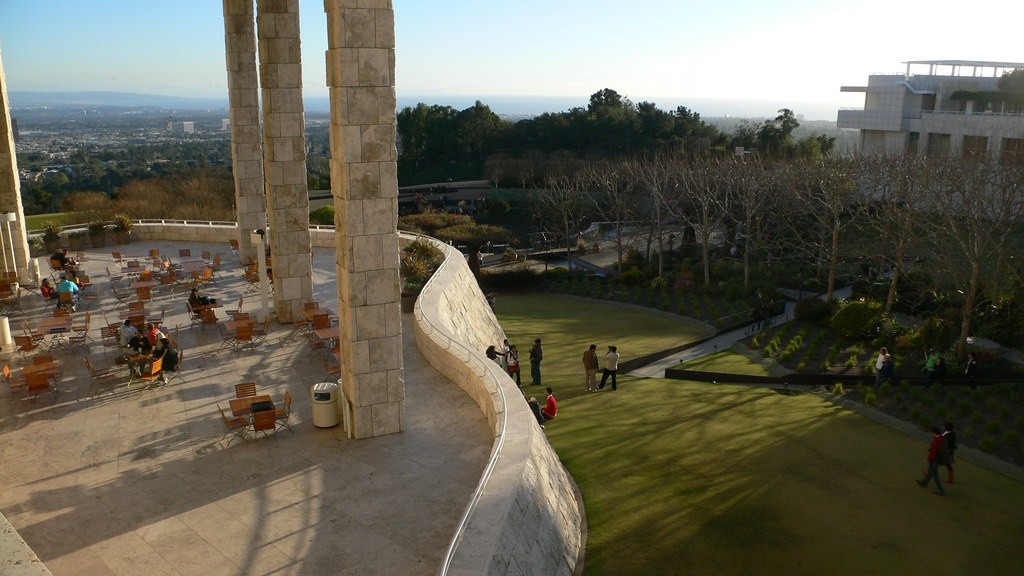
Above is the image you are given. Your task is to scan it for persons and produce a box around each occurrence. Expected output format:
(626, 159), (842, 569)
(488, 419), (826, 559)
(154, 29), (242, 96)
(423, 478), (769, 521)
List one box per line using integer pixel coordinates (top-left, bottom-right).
(506, 345), (524, 388)
(583, 344), (599, 392)
(541, 387), (558, 420)
(528, 397), (545, 426)
(485, 346), (505, 368)
(115, 318), (185, 383)
(528, 338), (544, 386)
(39, 246), (84, 312)
(873, 341), (984, 395)
(476, 250), (484, 269)
(916, 425), (948, 496)
(596, 346), (619, 390)
(922, 421), (957, 485)
(500, 338), (511, 374)
(187, 287), (205, 315)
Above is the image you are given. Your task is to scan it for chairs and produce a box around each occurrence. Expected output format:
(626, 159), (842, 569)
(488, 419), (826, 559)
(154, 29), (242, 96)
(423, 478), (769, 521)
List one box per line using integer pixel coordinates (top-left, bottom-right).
(7, 237), (342, 437)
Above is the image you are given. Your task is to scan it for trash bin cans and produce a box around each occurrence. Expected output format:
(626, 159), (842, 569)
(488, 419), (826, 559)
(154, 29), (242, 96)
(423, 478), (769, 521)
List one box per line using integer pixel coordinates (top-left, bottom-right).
(310, 382), (341, 429)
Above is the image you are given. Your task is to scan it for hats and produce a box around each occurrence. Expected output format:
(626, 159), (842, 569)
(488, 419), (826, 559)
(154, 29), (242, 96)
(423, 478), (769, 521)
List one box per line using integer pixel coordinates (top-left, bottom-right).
(528, 397), (539, 402)
(60, 276), (66, 279)
(191, 288), (198, 291)
(534, 339), (540, 342)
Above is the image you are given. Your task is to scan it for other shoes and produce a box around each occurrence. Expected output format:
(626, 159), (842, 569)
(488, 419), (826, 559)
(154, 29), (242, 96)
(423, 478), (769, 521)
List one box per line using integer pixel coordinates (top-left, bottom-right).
(517, 382), (542, 388)
(934, 491), (944, 496)
(584, 386), (617, 392)
(916, 479), (921, 487)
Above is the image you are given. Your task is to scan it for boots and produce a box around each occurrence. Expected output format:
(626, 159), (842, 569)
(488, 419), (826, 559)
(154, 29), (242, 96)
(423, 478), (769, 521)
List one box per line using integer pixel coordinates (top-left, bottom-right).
(944, 471), (954, 484)
